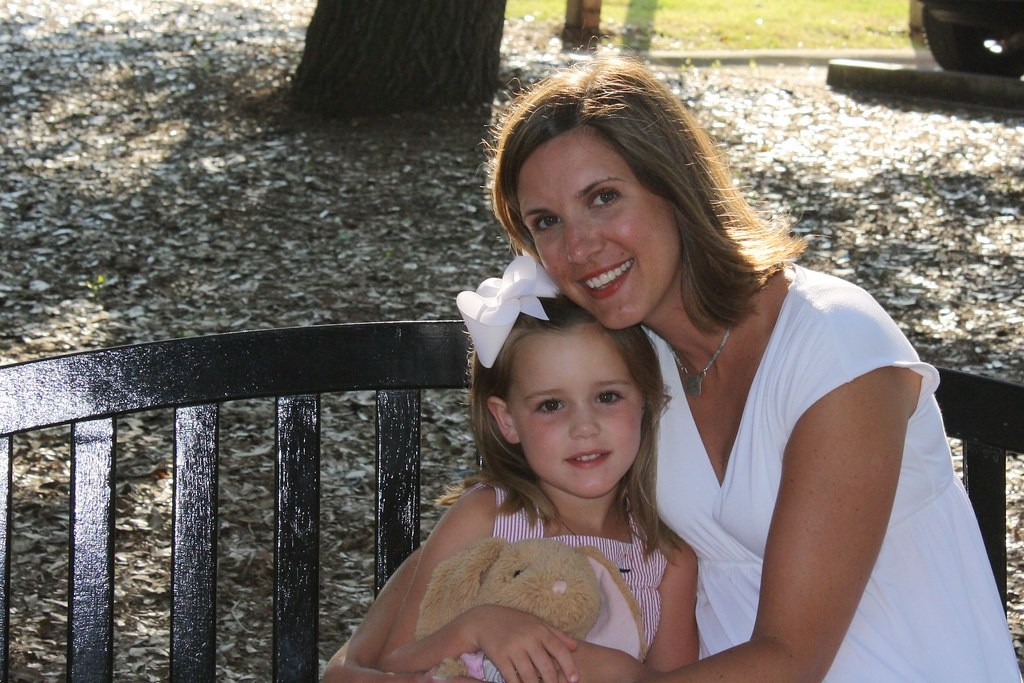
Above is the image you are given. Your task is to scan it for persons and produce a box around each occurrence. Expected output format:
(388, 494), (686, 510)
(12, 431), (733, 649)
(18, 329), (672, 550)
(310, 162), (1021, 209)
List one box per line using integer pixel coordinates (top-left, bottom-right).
(378, 254), (699, 683)
(318, 52), (1023, 683)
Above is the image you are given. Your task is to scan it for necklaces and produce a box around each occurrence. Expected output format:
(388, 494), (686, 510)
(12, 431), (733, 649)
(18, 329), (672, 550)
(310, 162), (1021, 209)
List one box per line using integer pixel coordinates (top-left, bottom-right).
(668, 324), (732, 394)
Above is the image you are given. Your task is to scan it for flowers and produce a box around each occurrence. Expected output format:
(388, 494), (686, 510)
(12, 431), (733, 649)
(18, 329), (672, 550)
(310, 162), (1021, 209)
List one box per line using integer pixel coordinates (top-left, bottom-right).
(457, 255), (560, 368)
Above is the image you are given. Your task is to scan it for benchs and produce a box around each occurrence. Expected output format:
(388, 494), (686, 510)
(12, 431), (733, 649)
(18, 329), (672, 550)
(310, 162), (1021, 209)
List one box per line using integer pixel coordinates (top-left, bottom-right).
(1, 320), (1023, 683)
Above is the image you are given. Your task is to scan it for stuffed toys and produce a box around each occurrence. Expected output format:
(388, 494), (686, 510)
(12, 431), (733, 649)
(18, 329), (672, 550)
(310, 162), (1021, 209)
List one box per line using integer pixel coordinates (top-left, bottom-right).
(416, 536), (649, 683)
